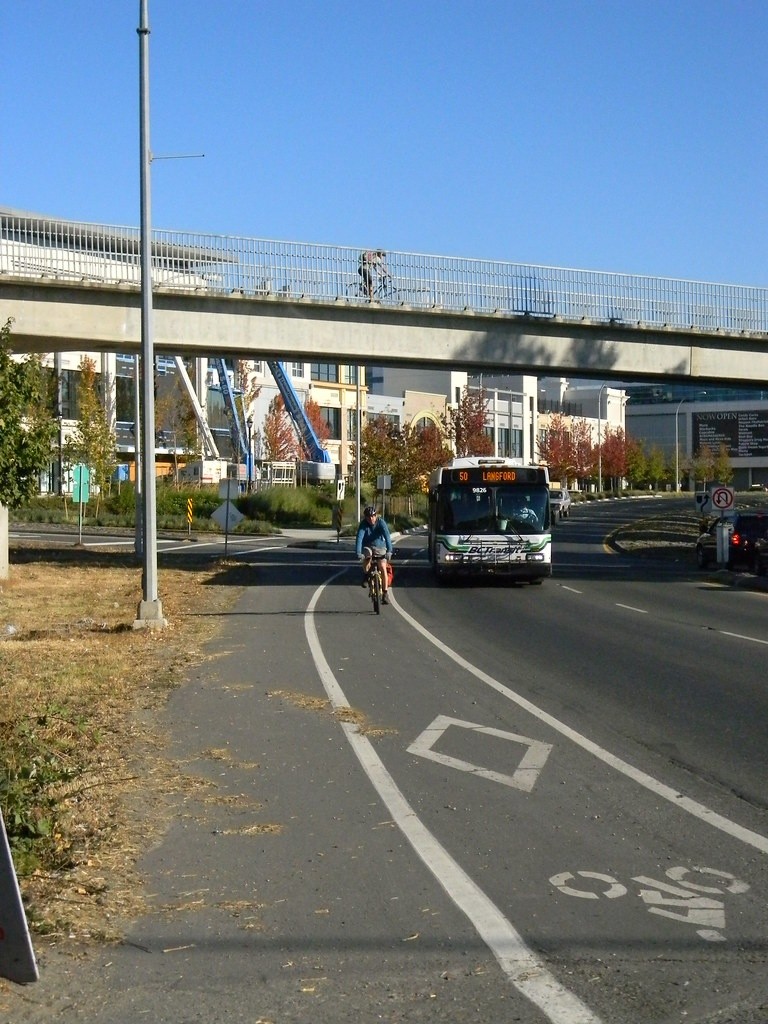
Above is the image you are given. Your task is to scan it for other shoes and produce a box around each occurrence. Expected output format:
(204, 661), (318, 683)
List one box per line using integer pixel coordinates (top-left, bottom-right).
(362, 574), (368, 588)
(382, 594), (389, 605)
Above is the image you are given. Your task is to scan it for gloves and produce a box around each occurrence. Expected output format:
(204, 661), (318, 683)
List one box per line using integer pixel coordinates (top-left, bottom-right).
(357, 554), (364, 561)
(384, 551), (391, 561)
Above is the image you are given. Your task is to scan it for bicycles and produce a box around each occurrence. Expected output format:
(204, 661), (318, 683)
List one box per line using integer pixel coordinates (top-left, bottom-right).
(365, 553), (388, 615)
(345, 274), (405, 306)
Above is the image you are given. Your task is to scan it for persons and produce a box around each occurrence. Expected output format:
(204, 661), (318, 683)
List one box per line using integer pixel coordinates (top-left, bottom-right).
(356, 506), (392, 605)
(358, 249), (388, 295)
(513, 496), (538, 523)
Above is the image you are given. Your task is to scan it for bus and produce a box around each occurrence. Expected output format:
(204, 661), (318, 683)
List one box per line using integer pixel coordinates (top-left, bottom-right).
(429, 455), (560, 581)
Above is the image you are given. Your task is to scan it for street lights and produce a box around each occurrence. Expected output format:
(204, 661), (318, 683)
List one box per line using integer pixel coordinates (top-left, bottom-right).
(676, 390), (707, 494)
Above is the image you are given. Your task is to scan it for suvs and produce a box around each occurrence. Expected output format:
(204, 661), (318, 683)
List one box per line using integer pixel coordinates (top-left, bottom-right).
(549, 487), (571, 519)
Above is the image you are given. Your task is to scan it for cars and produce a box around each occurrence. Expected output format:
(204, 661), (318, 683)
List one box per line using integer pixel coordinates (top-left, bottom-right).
(696, 511), (768, 575)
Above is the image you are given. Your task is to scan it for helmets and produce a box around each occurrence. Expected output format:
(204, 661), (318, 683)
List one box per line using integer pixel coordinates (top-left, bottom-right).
(364, 507), (376, 516)
